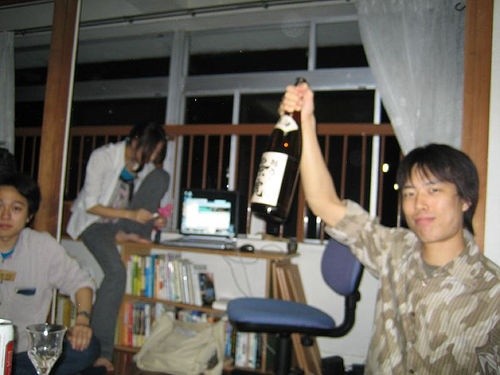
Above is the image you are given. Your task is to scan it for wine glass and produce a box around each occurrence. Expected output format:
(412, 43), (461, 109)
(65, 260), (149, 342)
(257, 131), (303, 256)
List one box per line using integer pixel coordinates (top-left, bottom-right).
(26, 324), (67, 375)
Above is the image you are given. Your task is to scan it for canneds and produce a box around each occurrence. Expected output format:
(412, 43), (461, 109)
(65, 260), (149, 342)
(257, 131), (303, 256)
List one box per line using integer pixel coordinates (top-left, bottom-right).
(0, 319), (14, 375)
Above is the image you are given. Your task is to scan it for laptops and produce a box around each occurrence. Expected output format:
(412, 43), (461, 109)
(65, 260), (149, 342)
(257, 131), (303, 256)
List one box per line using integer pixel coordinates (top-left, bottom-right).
(163, 187), (240, 250)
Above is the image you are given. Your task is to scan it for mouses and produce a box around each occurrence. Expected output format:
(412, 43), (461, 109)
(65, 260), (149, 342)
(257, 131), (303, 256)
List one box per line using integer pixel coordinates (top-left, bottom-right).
(239, 244), (254, 252)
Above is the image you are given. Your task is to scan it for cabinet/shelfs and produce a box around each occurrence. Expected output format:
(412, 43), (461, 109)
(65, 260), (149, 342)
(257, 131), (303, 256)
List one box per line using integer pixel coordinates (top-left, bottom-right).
(114, 241), (301, 375)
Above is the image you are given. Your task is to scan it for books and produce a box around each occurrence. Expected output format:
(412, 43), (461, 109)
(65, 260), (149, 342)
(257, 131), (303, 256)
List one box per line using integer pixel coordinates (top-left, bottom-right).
(126, 249), (216, 307)
(125, 302), (231, 358)
(236, 331), (260, 369)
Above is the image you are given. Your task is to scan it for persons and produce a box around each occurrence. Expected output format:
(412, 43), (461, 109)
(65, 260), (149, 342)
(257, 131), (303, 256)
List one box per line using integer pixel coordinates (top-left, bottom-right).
(0, 171), (96, 374)
(278, 80), (500, 375)
(66, 120), (171, 371)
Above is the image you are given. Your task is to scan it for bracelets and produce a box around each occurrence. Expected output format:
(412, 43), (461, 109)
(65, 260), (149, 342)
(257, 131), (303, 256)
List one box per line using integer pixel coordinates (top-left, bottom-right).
(76, 311), (91, 318)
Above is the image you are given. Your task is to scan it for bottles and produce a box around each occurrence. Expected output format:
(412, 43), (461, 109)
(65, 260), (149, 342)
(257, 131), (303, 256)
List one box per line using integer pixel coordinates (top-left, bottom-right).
(251, 77), (307, 223)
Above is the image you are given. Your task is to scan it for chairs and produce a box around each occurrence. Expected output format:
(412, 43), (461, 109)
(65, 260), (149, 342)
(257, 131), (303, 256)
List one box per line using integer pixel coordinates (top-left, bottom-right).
(228, 239), (366, 375)
